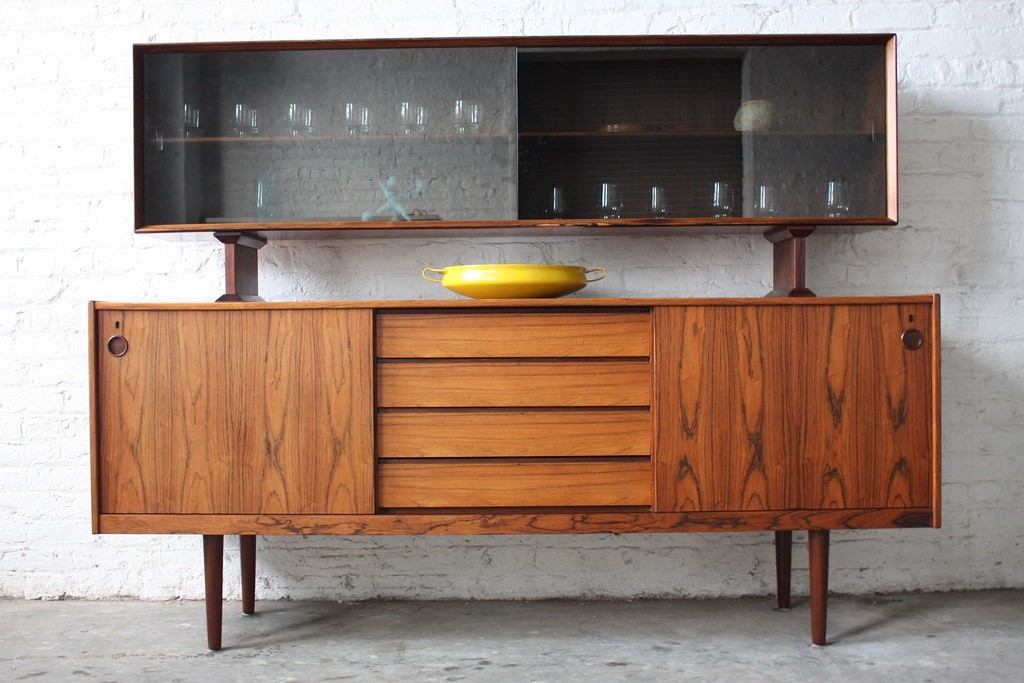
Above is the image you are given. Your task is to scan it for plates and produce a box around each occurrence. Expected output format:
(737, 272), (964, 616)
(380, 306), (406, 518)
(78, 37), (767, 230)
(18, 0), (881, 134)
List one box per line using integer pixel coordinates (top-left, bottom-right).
(593, 124), (641, 132)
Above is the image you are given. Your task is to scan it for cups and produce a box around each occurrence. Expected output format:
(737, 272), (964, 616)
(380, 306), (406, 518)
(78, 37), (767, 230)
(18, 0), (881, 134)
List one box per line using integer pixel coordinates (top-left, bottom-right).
(235, 103), (260, 137)
(182, 102), (203, 137)
(754, 186), (780, 217)
(287, 103), (315, 136)
(649, 186), (672, 218)
(596, 182), (623, 219)
(401, 101), (428, 134)
(453, 100), (482, 133)
(711, 181), (739, 217)
(825, 181), (850, 217)
(345, 102), (372, 135)
(544, 186), (568, 219)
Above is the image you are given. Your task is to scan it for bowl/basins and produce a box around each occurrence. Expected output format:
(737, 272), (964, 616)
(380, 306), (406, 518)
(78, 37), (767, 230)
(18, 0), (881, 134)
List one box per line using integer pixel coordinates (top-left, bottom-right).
(734, 100), (784, 131)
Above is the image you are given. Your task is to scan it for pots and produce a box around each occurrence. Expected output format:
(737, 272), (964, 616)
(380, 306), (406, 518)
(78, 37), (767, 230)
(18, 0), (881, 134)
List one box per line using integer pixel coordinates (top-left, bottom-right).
(421, 265), (606, 298)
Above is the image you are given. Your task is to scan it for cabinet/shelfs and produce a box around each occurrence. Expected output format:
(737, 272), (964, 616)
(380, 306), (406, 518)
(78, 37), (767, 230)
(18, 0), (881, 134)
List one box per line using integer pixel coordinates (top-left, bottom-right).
(89, 292), (941, 653)
(132, 33), (900, 302)
